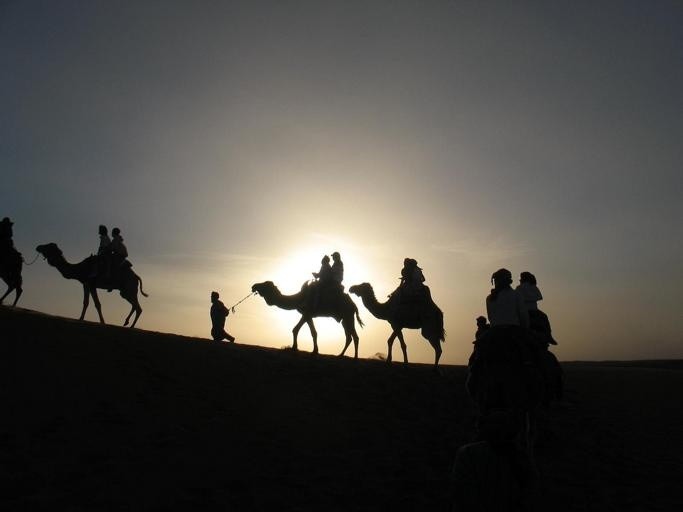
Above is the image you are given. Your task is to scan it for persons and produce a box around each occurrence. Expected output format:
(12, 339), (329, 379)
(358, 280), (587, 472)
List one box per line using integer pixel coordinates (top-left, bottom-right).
(210, 291), (235, 343)
(471, 269), (557, 345)
(97, 225), (112, 255)
(312, 255), (331, 282)
(0, 217), (15, 247)
(331, 252), (343, 281)
(112, 227), (128, 264)
(398, 258), (425, 286)
